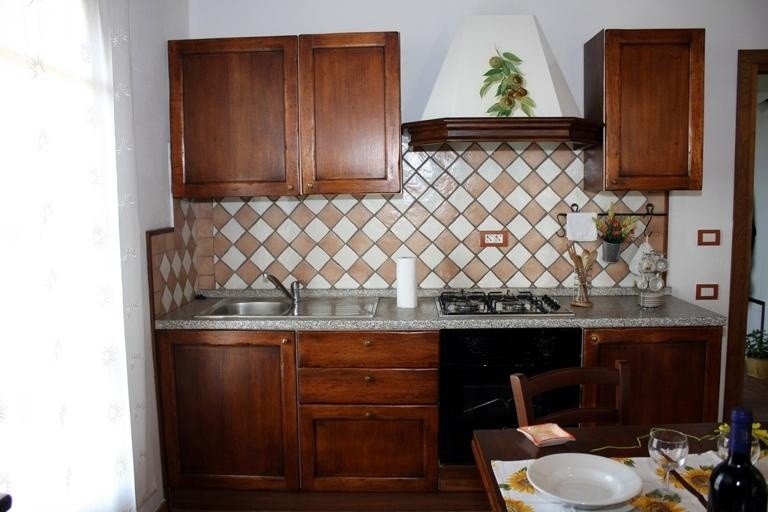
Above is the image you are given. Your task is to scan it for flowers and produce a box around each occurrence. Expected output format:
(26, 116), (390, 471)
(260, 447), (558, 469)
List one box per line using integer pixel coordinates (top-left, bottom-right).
(591, 201), (640, 242)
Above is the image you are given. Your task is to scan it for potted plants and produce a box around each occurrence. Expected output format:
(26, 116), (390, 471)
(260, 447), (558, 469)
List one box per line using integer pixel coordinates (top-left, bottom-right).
(745, 328), (768, 379)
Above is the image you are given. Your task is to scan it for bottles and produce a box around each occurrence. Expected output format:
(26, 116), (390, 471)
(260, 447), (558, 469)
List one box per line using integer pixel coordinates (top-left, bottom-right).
(709, 408), (767, 512)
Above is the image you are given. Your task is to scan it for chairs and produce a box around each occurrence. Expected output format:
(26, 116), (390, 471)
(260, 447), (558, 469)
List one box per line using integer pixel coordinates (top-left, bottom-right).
(509, 359), (631, 428)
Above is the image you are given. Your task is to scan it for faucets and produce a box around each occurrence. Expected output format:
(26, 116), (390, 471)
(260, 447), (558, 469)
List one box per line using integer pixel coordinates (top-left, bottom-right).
(262, 271), (306, 304)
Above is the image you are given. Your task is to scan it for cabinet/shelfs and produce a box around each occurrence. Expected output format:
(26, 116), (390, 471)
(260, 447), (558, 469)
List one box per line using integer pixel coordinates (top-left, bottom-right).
(167, 31), (404, 195)
(582, 28), (705, 192)
(578, 324), (723, 427)
(155, 330), (297, 492)
(298, 331), (440, 493)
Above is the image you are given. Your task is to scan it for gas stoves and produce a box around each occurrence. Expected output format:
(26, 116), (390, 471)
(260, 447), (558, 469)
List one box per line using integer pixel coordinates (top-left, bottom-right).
(434, 290), (576, 317)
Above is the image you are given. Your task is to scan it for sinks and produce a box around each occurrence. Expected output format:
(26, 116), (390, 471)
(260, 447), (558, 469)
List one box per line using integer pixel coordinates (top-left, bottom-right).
(209, 301), (293, 316)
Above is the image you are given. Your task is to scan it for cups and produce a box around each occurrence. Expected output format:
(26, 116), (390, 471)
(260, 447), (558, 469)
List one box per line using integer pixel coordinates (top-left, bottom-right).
(637, 248), (668, 292)
(716, 433), (760, 464)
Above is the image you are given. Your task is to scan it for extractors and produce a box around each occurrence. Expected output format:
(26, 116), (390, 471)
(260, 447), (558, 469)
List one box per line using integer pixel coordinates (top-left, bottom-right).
(402, 14), (598, 153)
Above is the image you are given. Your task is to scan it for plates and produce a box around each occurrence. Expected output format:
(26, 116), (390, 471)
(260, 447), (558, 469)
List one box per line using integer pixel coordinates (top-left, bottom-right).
(527, 451), (643, 509)
(515, 420), (576, 448)
(638, 290), (664, 308)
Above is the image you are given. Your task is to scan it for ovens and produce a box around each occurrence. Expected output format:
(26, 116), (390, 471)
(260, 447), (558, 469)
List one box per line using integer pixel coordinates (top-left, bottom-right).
(437, 328), (581, 490)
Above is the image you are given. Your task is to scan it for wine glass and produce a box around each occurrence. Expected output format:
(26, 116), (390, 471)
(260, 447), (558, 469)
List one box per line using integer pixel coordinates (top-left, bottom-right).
(647, 429), (691, 500)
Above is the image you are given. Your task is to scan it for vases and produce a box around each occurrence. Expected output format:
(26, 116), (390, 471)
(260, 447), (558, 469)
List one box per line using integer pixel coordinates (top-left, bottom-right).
(602, 242), (621, 264)
